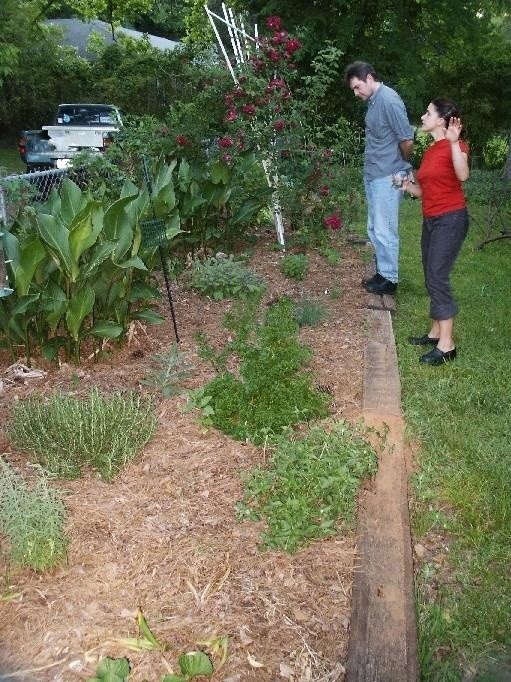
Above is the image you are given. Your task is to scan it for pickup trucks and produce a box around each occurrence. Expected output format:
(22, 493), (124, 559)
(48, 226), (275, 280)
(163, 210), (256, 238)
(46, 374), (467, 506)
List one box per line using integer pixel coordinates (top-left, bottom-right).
(20, 104), (124, 168)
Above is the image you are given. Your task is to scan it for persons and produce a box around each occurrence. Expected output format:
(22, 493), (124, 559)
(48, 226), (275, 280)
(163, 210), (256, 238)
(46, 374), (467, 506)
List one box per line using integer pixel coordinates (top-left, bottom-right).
(346, 62), (414, 293)
(400, 98), (473, 366)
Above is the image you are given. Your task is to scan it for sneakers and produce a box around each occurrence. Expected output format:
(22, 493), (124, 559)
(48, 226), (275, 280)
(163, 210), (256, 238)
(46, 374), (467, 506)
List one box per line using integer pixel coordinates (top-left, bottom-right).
(362, 273), (397, 295)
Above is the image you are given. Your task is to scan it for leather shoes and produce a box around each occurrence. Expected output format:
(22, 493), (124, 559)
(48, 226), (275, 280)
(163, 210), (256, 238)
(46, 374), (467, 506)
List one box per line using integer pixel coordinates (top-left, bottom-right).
(409, 334), (457, 365)
